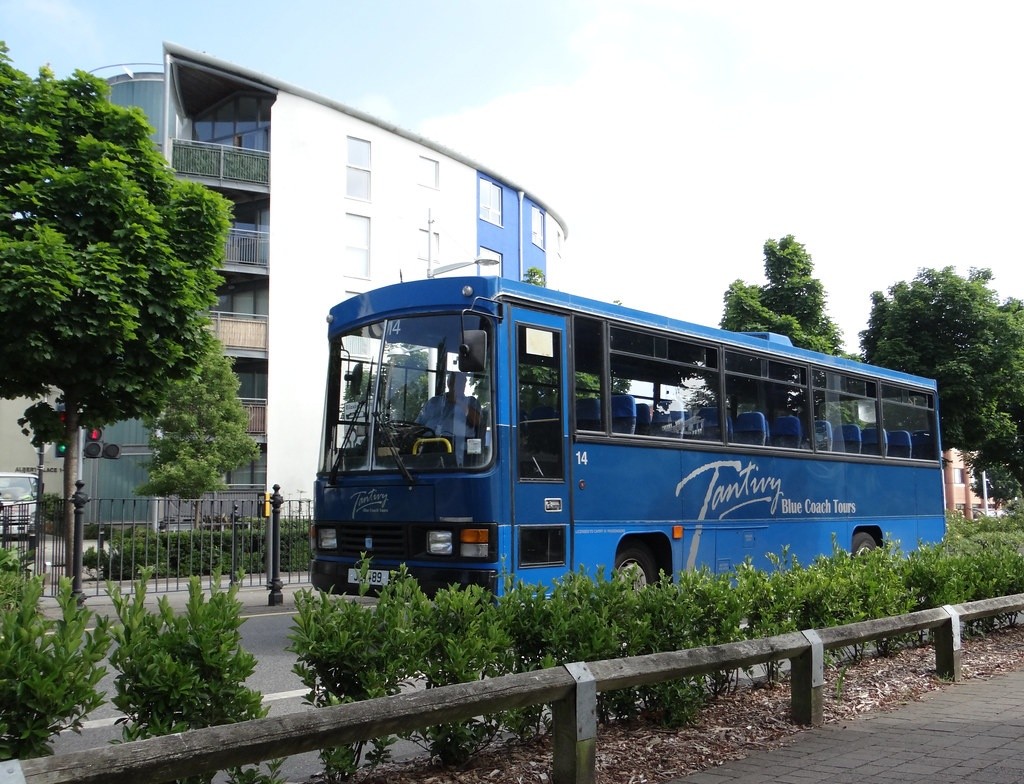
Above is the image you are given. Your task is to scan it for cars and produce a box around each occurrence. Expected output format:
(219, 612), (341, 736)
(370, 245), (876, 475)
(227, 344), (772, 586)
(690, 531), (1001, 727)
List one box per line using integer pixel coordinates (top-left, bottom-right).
(972, 508), (996, 520)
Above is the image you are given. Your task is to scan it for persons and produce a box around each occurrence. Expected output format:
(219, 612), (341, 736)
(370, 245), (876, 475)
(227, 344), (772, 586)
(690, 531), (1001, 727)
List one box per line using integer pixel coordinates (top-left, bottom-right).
(413, 372), (481, 468)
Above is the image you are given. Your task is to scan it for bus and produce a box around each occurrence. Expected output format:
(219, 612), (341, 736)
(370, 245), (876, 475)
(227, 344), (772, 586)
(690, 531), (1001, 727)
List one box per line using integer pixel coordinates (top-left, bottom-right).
(309, 275), (946, 610)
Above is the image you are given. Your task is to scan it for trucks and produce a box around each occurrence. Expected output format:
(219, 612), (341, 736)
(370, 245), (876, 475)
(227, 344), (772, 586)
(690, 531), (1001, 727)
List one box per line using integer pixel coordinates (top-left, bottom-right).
(0, 473), (44, 538)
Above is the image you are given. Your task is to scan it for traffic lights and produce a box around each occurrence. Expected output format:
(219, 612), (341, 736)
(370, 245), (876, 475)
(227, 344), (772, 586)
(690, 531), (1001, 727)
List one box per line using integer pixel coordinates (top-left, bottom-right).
(55, 411), (67, 458)
(83, 425), (123, 459)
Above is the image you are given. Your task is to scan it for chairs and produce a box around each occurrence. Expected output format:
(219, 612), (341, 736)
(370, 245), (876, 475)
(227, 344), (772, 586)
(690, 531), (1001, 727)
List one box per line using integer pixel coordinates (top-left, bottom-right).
(510, 396), (936, 466)
